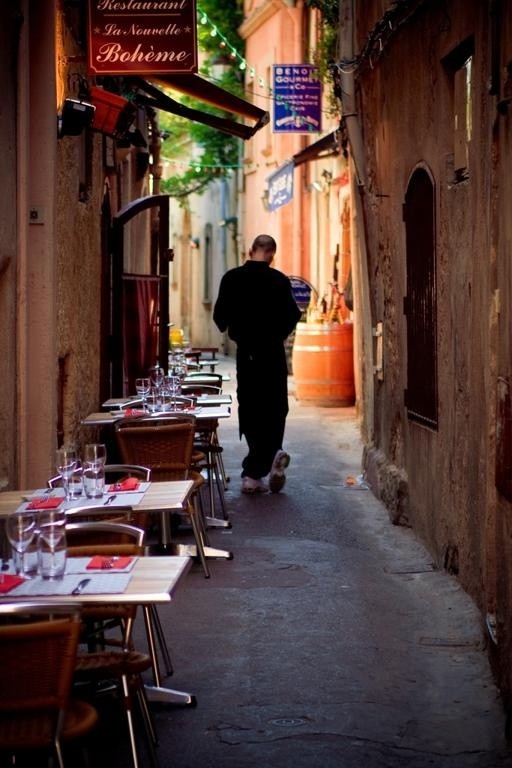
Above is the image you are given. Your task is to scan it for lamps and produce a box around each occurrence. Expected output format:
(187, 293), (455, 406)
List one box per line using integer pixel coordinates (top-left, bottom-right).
(218, 217), (237, 239)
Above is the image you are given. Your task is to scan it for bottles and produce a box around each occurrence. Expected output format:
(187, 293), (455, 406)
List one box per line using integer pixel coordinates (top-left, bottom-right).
(317, 288), (327, 324)
(306, 290), (318, 324)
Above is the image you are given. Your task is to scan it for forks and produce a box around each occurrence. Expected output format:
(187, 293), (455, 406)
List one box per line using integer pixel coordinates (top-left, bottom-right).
(29, 495), (49, 508)
(101, 556), (122, 571)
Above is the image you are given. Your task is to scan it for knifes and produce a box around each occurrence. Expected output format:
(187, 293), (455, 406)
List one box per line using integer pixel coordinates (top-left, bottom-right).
(103, 495), (118, 505)
(72, 577), (90, 596)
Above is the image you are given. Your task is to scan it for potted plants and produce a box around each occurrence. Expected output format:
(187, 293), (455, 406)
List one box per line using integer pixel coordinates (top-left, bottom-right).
(87, 76), (140, 141)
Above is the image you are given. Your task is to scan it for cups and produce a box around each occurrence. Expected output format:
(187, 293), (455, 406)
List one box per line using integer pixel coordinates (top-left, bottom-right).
(81, 464), (108, 500)
(36, 531), (68, 581)
(62, 469), (82, 501)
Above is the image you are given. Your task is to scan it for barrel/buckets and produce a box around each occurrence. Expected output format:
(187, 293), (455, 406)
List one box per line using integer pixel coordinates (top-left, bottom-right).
(292, 320), (355, 408)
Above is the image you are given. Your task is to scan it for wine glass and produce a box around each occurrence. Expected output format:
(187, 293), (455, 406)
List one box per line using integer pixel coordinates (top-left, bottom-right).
(6, 512), (35, 580)
(170, 341), (192, 384)
(54, 449), (79, 501)
(36, 508), (68, 571)
(84, 443), (107, 495)
(135, 368), (180, 417)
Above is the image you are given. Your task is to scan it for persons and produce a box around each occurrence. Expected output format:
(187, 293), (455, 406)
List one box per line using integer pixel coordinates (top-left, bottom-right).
(212, 235), (301, 493)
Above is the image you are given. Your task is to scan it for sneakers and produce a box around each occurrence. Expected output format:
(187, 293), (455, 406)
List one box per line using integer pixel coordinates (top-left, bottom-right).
(269, 449), (290, 493)
(241, 475), (271, 494)
